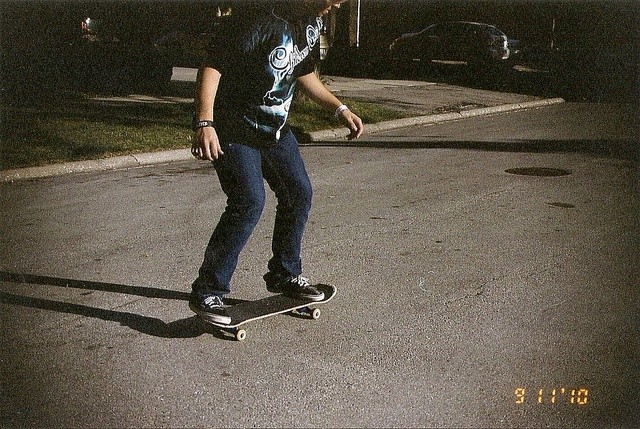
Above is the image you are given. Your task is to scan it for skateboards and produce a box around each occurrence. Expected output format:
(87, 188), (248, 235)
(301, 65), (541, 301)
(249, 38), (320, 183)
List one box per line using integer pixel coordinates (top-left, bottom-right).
(201, 283), (337, 341)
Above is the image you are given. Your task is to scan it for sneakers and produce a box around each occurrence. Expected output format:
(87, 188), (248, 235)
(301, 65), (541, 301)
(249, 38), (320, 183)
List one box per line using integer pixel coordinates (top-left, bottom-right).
(189, 289), (232, 325)
(267, 276), (324, 302)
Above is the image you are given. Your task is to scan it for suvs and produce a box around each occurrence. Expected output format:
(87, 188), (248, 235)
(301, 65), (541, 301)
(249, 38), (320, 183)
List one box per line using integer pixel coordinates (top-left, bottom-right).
(390, 21), (510, 70)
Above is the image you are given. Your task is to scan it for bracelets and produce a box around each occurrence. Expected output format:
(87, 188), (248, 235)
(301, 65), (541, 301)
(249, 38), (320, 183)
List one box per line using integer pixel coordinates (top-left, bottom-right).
(334, 105), (349, 117)
(192, 120), (216, 131)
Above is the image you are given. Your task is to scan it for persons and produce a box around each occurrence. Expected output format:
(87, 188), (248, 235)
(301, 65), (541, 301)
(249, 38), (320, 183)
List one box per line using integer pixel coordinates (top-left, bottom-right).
(189, 0), (363, 324)
